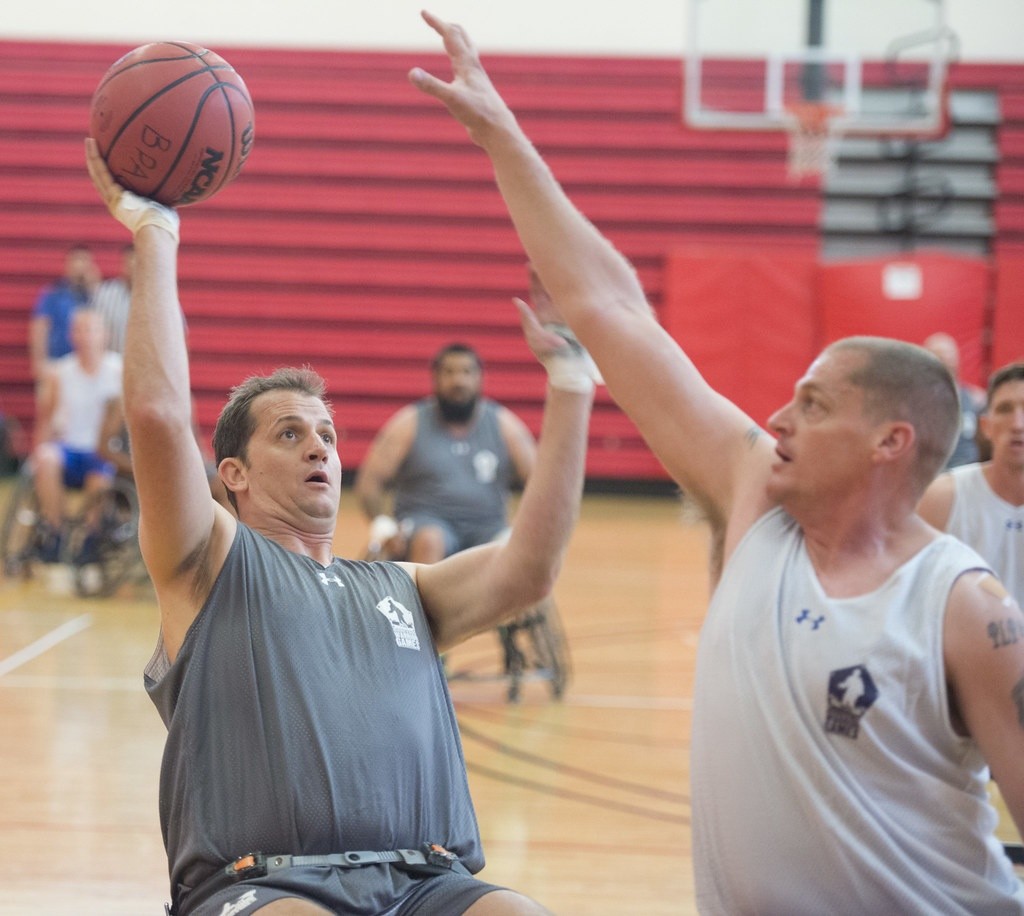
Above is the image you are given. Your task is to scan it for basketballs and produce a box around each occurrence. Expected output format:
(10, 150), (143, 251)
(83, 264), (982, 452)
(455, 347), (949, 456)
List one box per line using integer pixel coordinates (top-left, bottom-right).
(89, 44), (257, 208)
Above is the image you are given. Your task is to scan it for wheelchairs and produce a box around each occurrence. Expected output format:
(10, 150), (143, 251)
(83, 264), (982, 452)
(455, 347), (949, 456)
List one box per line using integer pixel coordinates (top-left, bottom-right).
(1, 441), (146, 602)
(365, 535), (569, 705)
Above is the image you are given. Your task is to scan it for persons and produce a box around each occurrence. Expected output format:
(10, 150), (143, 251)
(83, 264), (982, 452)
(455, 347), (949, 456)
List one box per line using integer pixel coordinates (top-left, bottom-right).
(358, 345), (541, 564)
(915, 334), (1024, 609)
(406, 11), (1024, 916)
(28, 308), (127, 562)
(90, 247), (135, 356)
(78, 134), (598, 916)
(30, 245), (94, 374)
